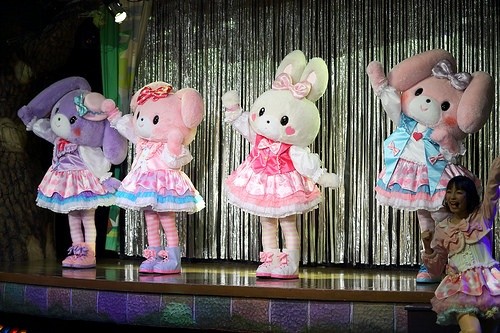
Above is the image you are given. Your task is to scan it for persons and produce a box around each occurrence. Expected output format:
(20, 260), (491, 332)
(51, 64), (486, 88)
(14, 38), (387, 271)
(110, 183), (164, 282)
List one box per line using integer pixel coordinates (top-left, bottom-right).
(420, 154), (500, 333)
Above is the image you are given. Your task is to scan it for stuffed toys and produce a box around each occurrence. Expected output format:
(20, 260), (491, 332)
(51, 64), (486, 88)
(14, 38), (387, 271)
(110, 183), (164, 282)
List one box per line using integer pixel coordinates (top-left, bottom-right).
(101, 82), (205, 273)
(222, 50), (340, 279)
(366, 49), (494, 282)
(18, 76), (128, 268)
